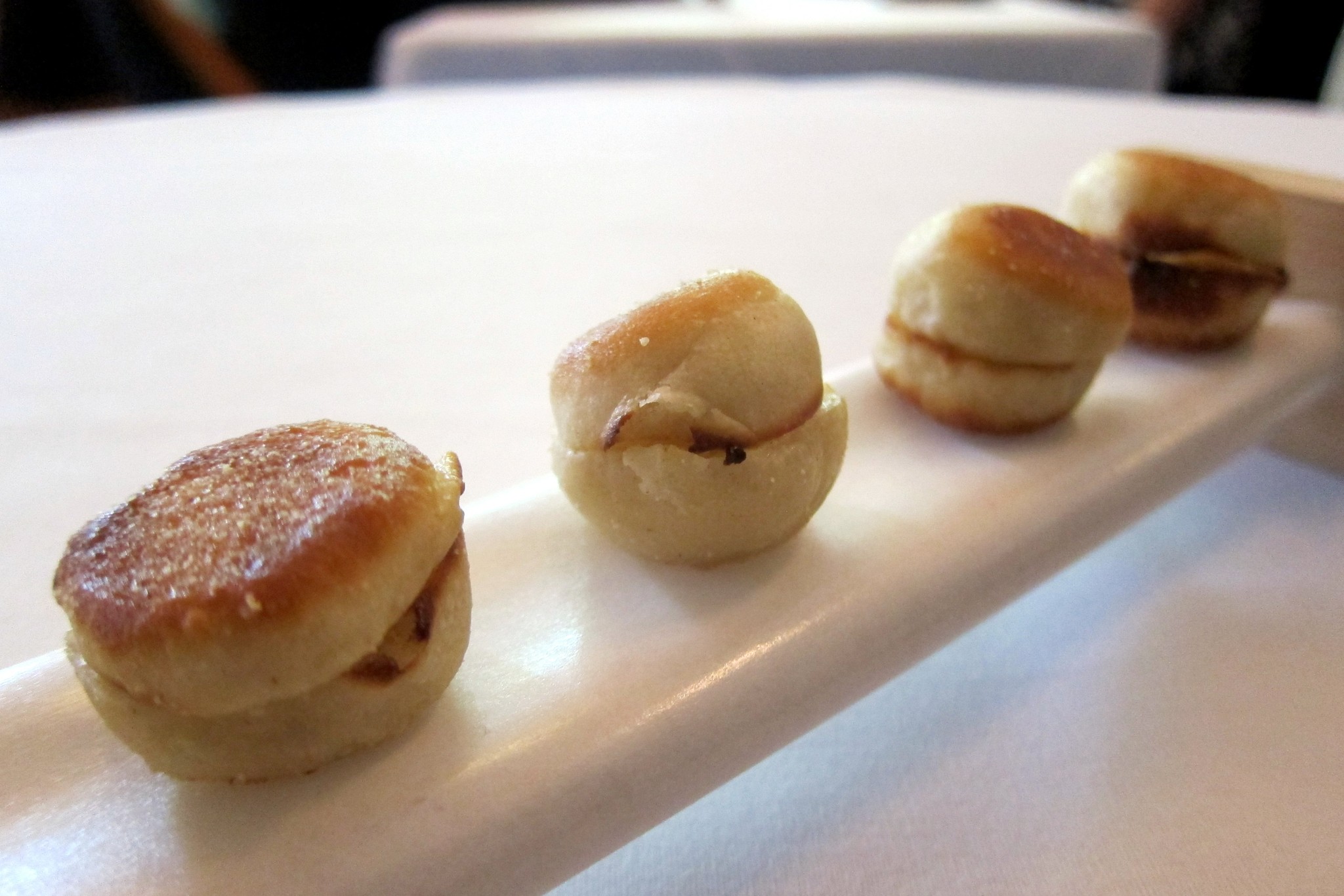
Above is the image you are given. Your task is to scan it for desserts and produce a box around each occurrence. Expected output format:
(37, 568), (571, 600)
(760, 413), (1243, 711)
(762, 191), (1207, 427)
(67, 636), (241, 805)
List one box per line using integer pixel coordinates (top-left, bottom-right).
(548, 269), (850, 565)
(54, 417), (472, 784)
(1057, 147), (1292, 352)
(869, 201), (1133, 436)
